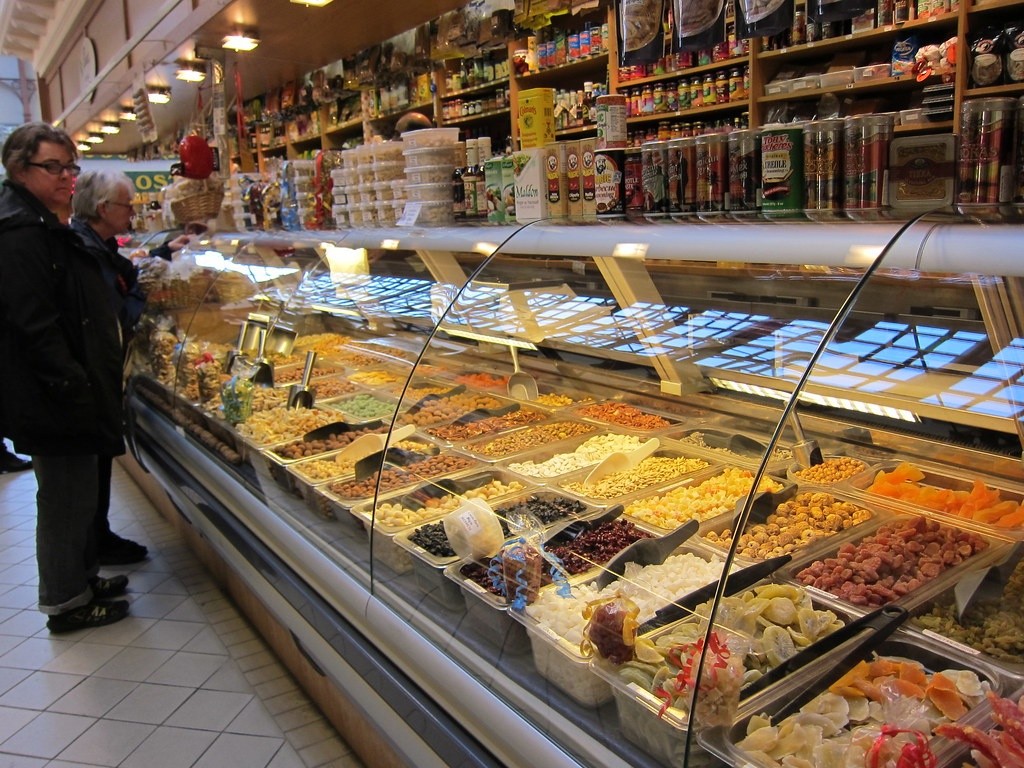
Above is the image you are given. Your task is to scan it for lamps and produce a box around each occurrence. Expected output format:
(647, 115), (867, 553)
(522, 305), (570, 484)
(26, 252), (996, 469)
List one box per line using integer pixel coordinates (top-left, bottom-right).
(174, 64), (207, 82)
(220, 30), (262, 51)
(76, 142), (91, 151)
(85, 134), (105, 144)
(147, 87), (171, 104)
(100, 122), (121, 134)
(119, 109), (136, 121)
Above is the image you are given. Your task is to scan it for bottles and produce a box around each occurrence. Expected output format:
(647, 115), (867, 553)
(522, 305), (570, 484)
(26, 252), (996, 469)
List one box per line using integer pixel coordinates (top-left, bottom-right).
(446, 58), (494, 92)
(465, 139), (478, 222)
(452, 142), (466, 222)
(476, 137), (493, 221)
(793, 11), (806, 45)
(620, 65), (750, 148)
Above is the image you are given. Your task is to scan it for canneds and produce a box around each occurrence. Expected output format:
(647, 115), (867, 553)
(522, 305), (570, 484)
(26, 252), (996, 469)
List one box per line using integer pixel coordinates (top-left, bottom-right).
(958, 95), (1024, 206)
(592, 92), (895, 220)
(442, 85), (510, 122)
(527, 23), (609, 73)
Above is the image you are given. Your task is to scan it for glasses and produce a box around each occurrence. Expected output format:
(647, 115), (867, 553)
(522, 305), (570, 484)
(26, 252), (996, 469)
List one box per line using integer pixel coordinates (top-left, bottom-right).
(28, 160), (82, 177)
(105, 198), (134, 213)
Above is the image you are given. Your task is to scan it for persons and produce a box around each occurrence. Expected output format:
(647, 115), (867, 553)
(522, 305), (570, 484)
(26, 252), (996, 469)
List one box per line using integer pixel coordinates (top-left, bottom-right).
(67, 168), (190, 564)
(394, 112), (432, 141)
(0, 124), (137, 633)
(0, 438), (33, 473)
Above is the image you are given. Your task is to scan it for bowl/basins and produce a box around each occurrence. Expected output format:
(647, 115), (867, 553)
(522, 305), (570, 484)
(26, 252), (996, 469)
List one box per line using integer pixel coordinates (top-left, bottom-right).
(143, 318), (1023, 768)
(294, 127), (459, 223)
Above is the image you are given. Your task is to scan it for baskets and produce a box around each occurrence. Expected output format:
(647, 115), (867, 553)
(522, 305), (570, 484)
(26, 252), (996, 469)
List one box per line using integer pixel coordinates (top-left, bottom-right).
(169, 179), (223, 220)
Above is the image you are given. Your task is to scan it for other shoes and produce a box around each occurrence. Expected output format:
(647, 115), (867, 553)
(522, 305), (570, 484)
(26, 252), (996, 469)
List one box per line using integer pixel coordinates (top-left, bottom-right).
(103, 532), (146, 565)
(0, 447), (32, 473)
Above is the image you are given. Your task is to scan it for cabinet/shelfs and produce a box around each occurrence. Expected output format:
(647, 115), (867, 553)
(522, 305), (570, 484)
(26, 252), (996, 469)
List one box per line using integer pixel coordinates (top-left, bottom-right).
(232, 0), (1024, 174)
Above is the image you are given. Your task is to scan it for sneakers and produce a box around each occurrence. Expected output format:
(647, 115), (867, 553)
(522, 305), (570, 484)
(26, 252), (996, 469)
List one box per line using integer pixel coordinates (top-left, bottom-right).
(93, 574), (128, 601)
(47, 601), (129, 637)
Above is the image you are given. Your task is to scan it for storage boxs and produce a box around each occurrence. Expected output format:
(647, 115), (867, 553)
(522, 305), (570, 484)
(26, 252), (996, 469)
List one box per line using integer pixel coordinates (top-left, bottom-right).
(877, 112), (900, 126)
(852, 63), (892, 83)
(819, 70), (854, 88)
(140, 342), (1024, 768)
(763, 81), (788, 96)
(228, 71), (599, 228)
(900, 109), (926, 125)
(787, 75), (818, 93)
(851, 8), (874, 34)
